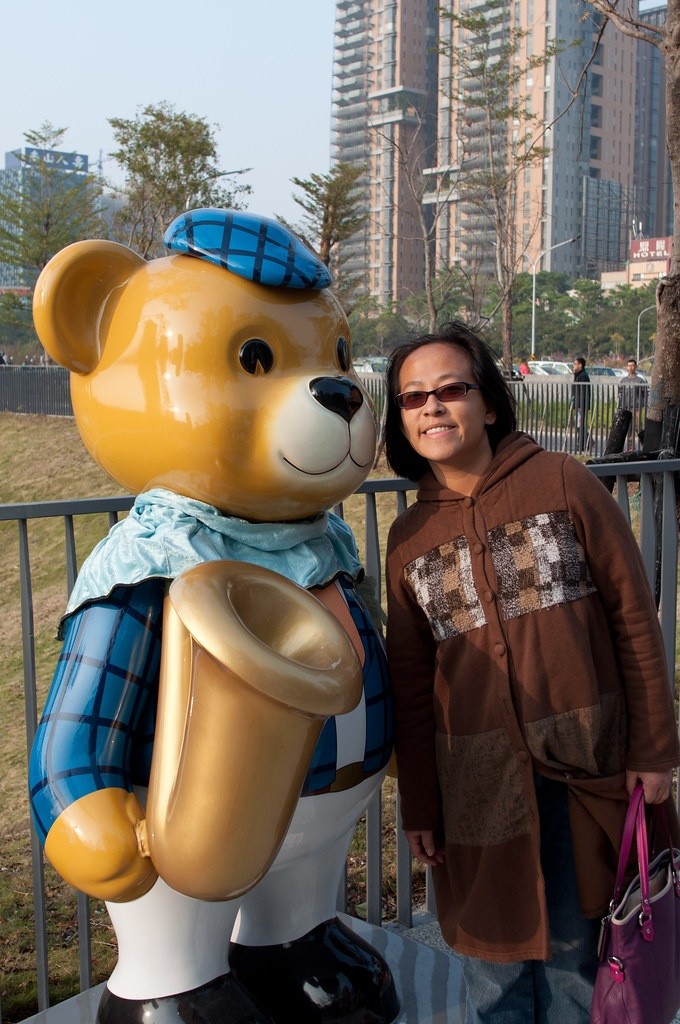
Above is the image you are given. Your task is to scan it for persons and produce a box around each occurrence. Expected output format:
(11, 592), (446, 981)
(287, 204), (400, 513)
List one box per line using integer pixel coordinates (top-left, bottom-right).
(23, 355), (53, 365)
(384, 323), (679, 1023)
(0, 351), (13, 365)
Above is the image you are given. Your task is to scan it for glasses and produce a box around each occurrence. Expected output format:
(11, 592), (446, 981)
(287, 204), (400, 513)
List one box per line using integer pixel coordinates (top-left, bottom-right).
(394, 381), (481, 409)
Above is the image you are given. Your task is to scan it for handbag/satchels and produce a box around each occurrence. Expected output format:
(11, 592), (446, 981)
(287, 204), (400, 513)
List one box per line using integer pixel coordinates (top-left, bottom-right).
(591, 784), (680, 1024)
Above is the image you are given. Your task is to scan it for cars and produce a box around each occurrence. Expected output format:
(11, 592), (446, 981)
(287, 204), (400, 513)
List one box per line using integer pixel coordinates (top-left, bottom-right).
(496, 360), (649, 379)
(352, 355), (392, 373)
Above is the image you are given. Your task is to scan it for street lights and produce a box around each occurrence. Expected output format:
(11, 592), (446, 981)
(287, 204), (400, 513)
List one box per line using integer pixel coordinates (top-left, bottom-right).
(531, 233), (582, 360)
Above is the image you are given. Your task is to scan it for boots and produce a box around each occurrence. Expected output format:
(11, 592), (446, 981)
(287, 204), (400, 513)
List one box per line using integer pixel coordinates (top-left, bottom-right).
(582, 430), (596, 448)
(577, 427), (584, 449)
